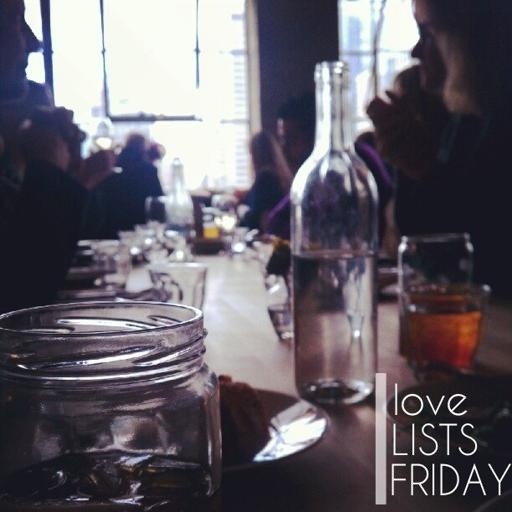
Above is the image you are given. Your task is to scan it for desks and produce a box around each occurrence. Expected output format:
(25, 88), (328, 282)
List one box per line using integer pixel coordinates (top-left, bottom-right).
(52, 227), (512, 512)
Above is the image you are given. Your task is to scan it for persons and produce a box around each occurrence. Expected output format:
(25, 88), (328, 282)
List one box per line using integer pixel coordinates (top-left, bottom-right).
(1, 2), (512, 418)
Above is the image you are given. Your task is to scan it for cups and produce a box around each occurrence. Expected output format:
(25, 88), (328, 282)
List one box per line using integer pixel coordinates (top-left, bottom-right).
(398, 229), (487, 379)
(148, 262), (207, 311)
(93, 242), (130, 290)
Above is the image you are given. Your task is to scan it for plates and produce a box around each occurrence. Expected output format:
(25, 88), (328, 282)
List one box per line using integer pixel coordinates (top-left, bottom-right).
(221, 389), (333, 475)
(389, 373), (511, 457)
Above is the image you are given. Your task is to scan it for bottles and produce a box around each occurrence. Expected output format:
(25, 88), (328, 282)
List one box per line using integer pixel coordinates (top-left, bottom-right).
(289, 60), (380, 409)
(0, 301), (223, 512)
(166, 160), (192, 243)
